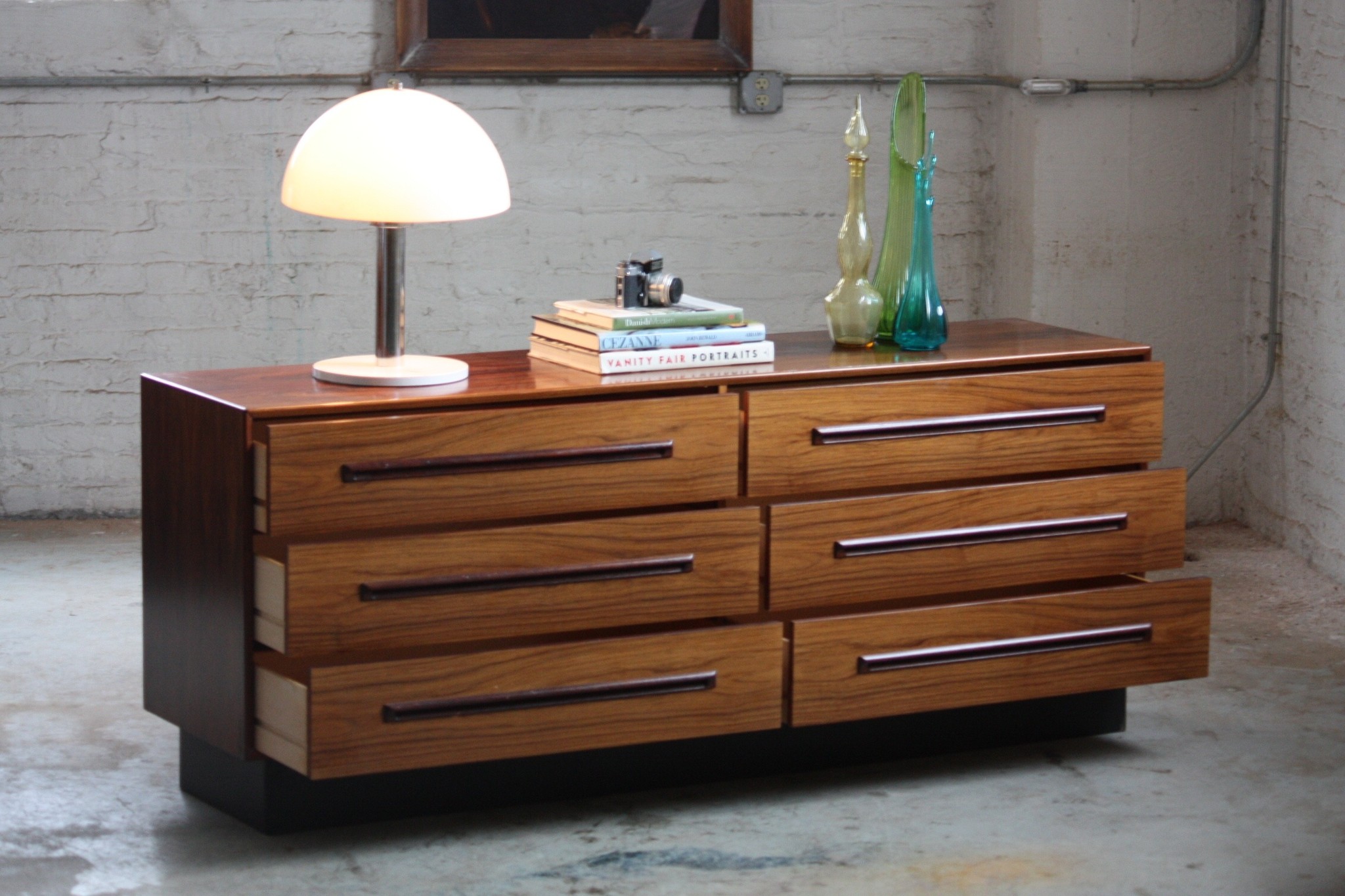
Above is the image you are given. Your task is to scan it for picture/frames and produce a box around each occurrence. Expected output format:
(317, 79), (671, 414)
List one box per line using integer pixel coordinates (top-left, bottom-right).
(395, 0), (753, 78)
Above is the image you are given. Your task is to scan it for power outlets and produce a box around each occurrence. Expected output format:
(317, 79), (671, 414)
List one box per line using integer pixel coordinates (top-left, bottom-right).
(734, 68), (784, 113)
(370, 69), (419, 91)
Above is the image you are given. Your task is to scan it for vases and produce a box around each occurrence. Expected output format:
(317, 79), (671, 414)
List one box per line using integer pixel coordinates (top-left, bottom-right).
(868, 69), (949, 352)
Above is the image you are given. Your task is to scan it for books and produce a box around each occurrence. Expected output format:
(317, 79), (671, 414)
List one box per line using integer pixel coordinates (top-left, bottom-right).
(526, 293), (775, 377)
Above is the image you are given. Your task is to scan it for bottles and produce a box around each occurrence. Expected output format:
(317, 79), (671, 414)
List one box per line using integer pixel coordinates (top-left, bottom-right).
(870, 72), (927, 340)
(892, 130), (947, 350)
(824, 93), (884, 347)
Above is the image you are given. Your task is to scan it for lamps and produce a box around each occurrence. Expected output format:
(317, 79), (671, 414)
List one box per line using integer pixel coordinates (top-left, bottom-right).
(281, 82), (510, 388)
(1020, 77), (1074, 97)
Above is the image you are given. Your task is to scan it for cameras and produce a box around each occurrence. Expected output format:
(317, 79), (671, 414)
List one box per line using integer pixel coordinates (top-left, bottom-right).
(615, 254), (684, 310)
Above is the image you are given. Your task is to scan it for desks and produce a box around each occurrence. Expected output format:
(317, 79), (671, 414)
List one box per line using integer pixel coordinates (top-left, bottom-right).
(139, 321), (1211, 839)
